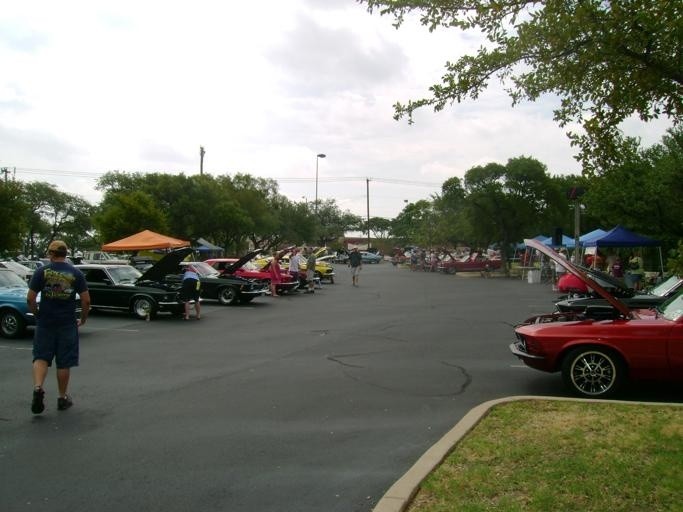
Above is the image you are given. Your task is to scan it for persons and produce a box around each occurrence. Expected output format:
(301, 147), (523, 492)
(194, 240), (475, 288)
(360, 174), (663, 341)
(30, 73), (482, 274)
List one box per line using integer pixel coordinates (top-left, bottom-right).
(303, 247), (315, 294)
(26, 240), (90, 414)
(541, 248), (645, 292)
(411, 249), (439, 273)
(270, 251), (281, 297)
(181, 271), (201, 321)
(348, 246), (362, 287)
(288, 250), (300, 292)
(482, 264), (491, 279)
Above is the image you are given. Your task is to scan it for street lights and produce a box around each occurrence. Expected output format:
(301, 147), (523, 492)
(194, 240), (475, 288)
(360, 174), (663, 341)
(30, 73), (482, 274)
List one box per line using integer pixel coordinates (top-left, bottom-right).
(313, 153), (326, 214)
(403, 200), (408, 215)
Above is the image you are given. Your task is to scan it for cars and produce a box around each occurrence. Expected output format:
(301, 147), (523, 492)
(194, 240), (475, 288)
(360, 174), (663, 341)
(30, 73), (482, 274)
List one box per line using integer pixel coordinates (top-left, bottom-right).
(400, 248), (502, 275)
(509, 238), (682, 398)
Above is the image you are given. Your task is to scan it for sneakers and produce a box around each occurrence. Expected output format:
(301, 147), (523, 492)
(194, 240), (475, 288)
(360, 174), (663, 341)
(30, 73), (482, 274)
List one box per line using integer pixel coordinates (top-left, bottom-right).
(304, 289), (317, 294)
(56, 395), (73, 410)
(30, 386), (45, 415)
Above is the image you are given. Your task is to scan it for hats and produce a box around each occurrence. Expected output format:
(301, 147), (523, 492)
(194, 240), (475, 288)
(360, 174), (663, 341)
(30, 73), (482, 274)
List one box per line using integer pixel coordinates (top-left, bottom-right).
(44, 239), (67, 257)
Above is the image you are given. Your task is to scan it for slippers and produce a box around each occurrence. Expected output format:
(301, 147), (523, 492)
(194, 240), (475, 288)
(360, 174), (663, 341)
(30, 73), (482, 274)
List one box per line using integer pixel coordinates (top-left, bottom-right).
(184, 315), (201, 322)
